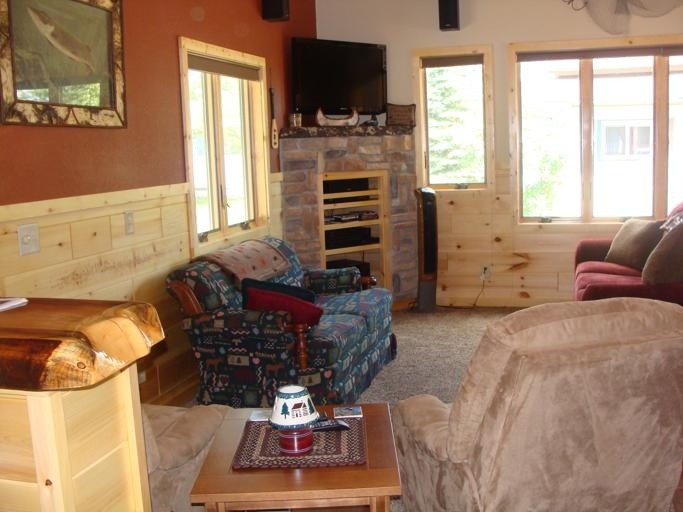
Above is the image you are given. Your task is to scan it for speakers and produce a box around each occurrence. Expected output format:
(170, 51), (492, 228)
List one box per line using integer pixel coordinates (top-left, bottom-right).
(413, 187), (438, 312)
(439, 1), (460, 31)
(261, 0), (289, 21)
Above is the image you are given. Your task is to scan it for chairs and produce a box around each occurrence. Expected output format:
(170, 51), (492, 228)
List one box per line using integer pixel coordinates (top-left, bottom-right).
(391, 296), (683, 512)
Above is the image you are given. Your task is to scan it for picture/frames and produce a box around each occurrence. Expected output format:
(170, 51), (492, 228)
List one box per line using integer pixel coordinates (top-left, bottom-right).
(0, 1), (128, 131)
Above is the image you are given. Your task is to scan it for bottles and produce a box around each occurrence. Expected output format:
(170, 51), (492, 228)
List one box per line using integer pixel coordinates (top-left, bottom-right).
(289, 103), (302, 127)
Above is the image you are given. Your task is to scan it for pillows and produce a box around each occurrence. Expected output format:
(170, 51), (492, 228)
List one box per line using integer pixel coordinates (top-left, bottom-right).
(238, 274), (324, 327)
(603, 217), (683, 287)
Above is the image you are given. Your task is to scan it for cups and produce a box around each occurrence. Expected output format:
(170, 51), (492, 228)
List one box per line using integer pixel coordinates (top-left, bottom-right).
(267, 382), (321, 457)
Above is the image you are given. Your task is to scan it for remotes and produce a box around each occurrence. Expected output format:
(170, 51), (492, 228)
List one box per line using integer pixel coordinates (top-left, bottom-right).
(309, 420), (351, 432)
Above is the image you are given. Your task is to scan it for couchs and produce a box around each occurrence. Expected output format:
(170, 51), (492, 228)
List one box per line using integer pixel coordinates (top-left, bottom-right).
(138, 403), (233, 512)
(564, 198), (682, 311)
(167, 229), (399, 415)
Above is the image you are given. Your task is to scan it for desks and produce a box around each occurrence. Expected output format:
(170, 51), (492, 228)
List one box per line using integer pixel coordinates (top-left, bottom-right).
(186, 396), (405, 512)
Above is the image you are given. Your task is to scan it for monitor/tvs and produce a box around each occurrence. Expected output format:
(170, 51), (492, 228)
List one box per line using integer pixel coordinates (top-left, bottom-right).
(290, 36), (388, 115)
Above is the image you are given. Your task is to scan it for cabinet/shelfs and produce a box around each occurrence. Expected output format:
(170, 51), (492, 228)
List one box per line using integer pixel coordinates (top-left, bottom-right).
(315, 169), (394, 292)
